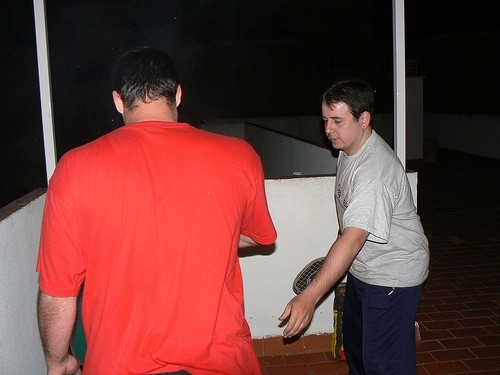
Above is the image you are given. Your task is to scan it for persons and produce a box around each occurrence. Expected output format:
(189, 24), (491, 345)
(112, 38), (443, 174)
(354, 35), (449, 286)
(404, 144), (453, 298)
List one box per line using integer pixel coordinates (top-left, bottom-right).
(37, 46), (278, 375)
(279, 78), (429, 375)
